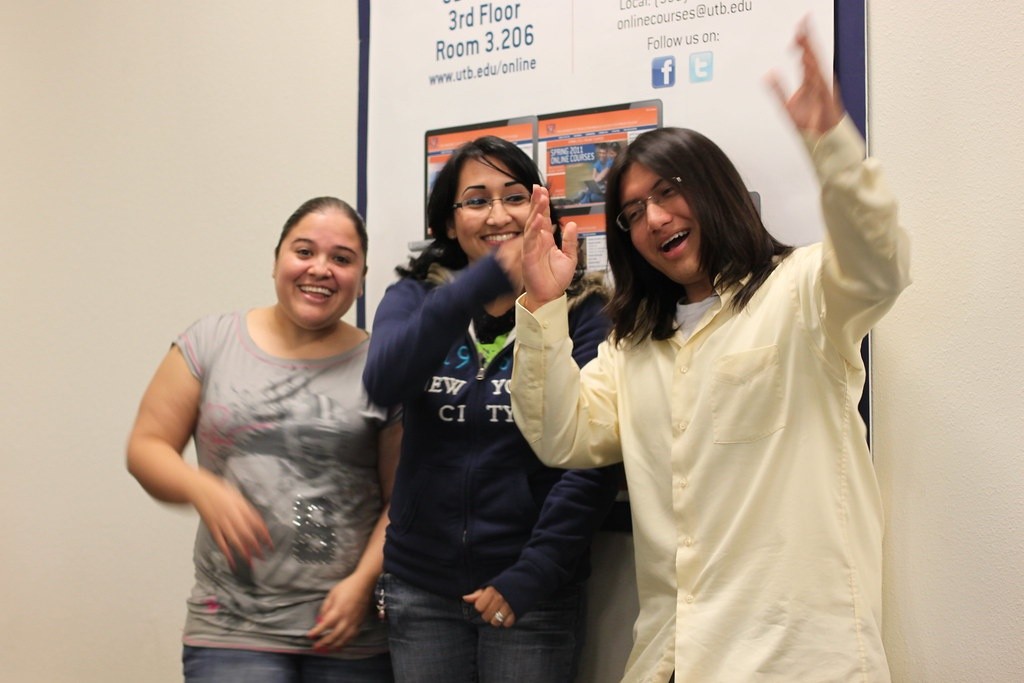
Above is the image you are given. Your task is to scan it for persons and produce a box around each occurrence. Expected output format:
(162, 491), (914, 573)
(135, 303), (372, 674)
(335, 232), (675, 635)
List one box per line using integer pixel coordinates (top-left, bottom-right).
(578, 142), (621, 204)
(508, 14), (913, 683)
(126, 196), (403, 683)
(362, 136), (622, 683)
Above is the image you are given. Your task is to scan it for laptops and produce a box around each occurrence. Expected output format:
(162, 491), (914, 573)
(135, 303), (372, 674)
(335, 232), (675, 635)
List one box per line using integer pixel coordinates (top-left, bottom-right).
(584, 179), (606, 195)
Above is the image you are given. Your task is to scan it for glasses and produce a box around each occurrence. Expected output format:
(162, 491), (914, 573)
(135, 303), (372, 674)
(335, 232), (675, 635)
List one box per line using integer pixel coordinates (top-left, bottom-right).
(616, 176), (684, 232)
(450, 188), (533, 216)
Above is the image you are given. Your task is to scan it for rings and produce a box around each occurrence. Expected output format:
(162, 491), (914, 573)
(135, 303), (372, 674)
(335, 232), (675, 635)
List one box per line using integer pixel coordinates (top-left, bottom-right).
(495, 611), (504, 623)
